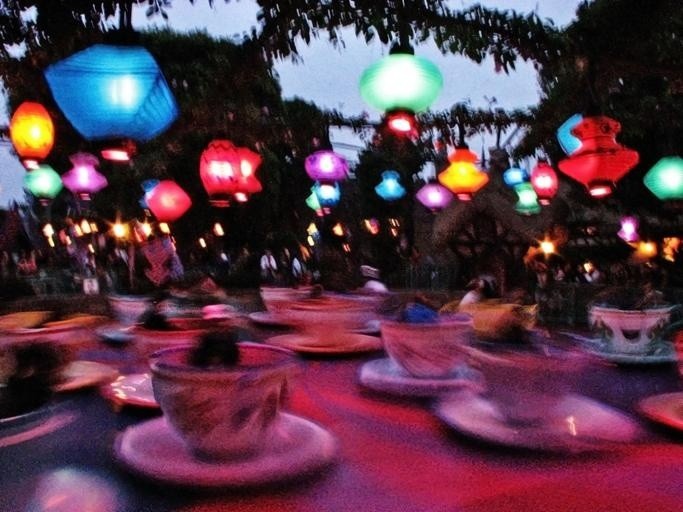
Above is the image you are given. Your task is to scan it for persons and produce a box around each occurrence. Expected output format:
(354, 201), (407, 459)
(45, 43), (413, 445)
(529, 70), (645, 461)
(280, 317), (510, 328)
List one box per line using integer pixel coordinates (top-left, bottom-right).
(2, 219), (682, 370)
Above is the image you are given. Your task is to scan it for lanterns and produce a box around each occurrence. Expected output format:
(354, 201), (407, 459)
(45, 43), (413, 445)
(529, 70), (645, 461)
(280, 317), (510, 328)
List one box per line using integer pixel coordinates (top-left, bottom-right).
(10, 46), (683, 222)
